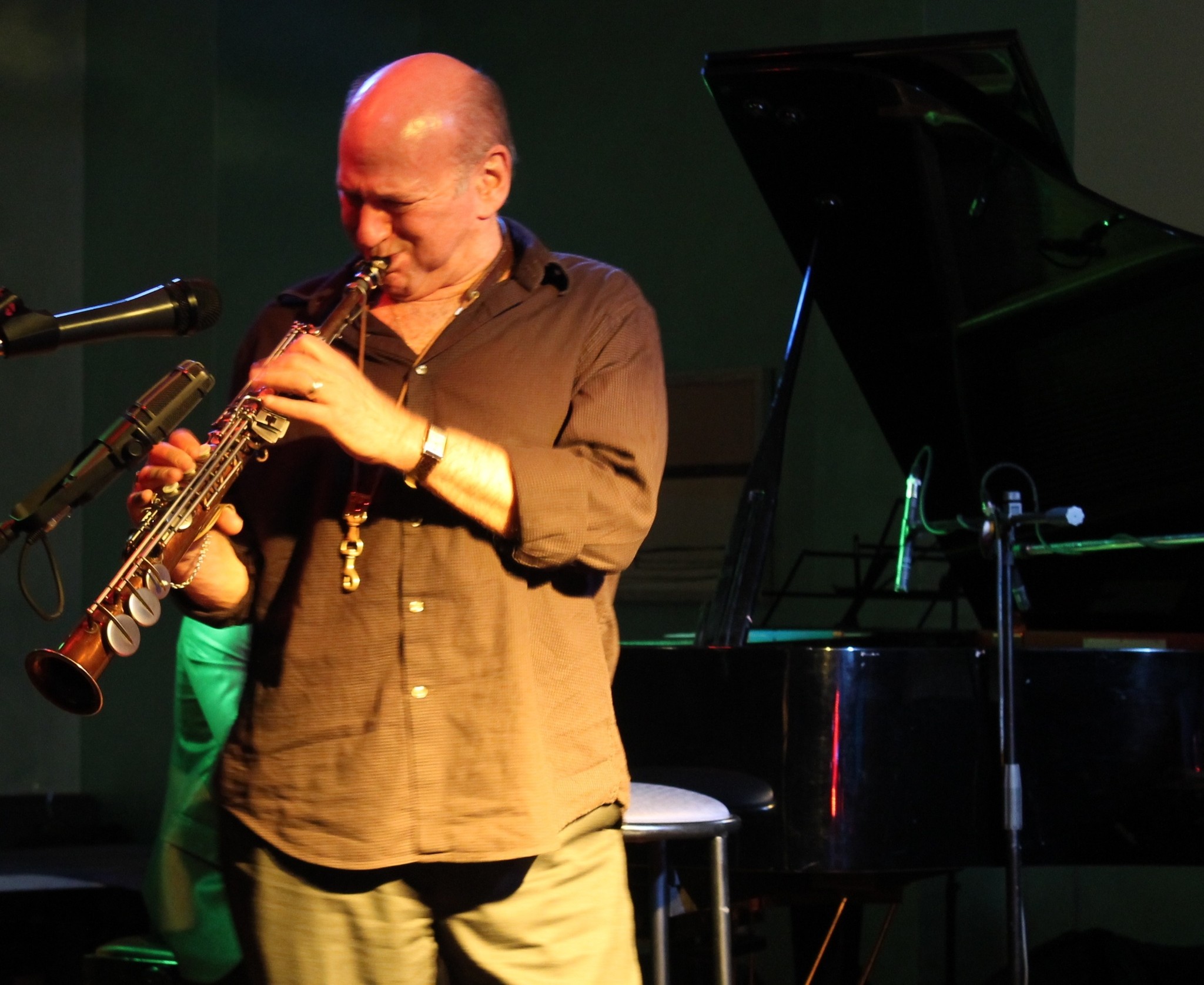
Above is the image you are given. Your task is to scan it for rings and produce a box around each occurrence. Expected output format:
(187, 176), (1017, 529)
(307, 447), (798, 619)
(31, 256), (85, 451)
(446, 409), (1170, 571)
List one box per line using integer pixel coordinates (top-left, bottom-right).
(307, 370), (326, 401)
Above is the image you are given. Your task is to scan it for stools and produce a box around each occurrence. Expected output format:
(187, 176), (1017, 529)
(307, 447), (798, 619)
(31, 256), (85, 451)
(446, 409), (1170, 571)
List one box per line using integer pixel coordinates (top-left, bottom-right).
(620, 768), (775, 985)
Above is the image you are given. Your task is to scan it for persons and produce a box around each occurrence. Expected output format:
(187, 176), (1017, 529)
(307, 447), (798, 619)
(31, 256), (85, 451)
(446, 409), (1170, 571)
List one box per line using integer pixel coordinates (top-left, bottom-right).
(152, 619), (252, 984)
(123, 48), (669, 985)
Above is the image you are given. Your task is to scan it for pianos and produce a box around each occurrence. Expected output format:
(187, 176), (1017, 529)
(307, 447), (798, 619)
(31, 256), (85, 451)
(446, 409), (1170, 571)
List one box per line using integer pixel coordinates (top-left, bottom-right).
(614, 24), (1202, 985)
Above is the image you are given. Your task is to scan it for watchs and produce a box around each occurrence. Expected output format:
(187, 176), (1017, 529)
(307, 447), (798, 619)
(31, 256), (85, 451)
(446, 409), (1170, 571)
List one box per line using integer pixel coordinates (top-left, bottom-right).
(403, 419), (449, 491)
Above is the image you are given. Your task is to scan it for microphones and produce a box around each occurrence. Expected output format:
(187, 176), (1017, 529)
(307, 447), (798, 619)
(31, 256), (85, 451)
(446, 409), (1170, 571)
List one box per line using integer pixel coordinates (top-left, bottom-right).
(894, 466), (921, 594)
(0, 277), (223, 359)
(16, 361), (217, 536)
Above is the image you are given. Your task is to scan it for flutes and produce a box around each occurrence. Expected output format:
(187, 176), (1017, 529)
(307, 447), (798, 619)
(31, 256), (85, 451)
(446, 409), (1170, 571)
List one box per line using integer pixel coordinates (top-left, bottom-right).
(24, 257), (388, 720)
